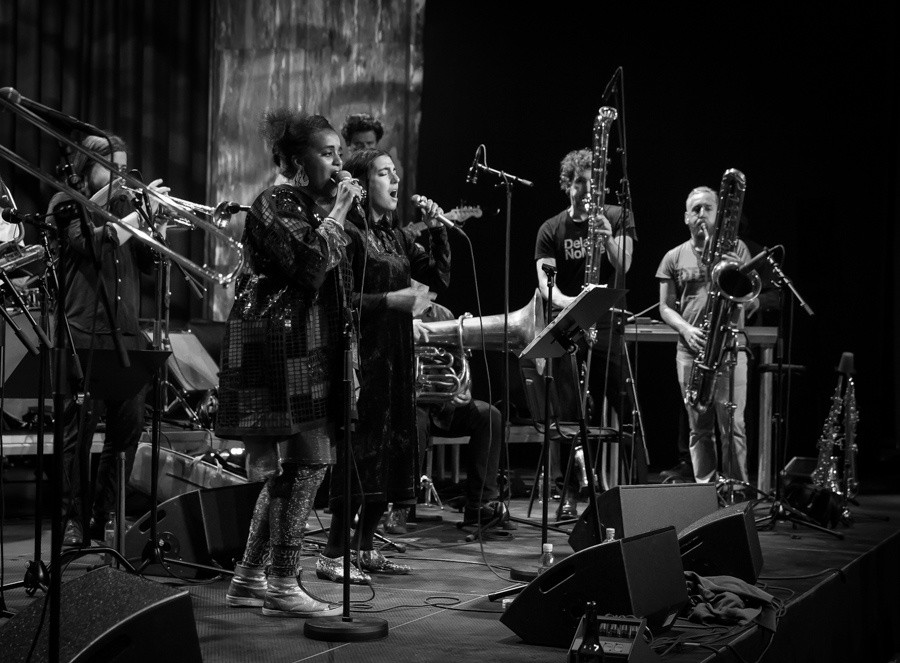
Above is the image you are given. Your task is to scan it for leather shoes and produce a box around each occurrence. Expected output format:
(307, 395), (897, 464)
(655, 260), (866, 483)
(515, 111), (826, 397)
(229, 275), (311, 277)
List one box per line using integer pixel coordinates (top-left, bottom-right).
(557, 491), (578, 519)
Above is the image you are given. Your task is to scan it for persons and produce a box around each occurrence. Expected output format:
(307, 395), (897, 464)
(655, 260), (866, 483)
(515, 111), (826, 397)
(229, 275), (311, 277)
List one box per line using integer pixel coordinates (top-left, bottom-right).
(46, 135), (171, 547)
(536, 150), (648, 519)
(215, 109), (362, 619)
(313, 111), (518, 584)
(0, 207), (29, 308)
(656, 186), (752, 491)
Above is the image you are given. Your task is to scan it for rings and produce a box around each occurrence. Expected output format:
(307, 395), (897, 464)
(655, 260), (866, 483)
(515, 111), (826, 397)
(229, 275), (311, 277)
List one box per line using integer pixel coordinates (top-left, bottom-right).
(350, 180), (356, 185)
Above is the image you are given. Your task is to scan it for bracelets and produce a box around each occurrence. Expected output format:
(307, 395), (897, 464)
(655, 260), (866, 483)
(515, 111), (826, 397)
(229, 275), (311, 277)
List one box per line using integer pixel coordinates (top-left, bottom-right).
(134, 209), (144, 229)
(324, 217), (345, 231)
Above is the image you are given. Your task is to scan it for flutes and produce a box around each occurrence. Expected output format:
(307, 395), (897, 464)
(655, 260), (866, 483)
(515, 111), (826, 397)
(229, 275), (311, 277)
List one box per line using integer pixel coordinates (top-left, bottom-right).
(810, 374), (860, 502)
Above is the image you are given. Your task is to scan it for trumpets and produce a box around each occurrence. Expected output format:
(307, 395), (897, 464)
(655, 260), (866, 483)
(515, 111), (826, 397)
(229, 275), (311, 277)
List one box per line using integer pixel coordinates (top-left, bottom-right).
(120, 183), (232, 228)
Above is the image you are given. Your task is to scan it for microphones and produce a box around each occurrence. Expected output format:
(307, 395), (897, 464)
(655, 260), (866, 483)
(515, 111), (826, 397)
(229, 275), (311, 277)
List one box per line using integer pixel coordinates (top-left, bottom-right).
(739, 245), (780, 275)
(0, 86), (106, 136)
(336, 170), (366, 218)
(411, 194), (465, 237)
(466, 147), (481, 185)
(224, 202), (251, 215)
(601, 68), (621, 102)
(2, 207), (57, 231)
(86, 177), (126, 212)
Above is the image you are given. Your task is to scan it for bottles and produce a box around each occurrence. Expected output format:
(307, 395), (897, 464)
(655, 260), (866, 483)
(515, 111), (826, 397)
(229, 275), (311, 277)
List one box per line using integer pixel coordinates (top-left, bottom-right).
(537, 544), (557, 573)
(603, 528), (615, 541)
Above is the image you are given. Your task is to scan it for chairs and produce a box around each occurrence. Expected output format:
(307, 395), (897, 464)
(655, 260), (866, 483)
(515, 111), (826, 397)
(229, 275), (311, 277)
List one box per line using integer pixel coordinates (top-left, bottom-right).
(522, 368), (618, 518)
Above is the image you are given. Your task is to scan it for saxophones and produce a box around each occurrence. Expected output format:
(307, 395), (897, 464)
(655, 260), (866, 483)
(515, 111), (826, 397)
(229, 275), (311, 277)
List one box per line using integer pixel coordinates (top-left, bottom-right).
(580, 105), (618, 290)
(683, 168), (764, 415)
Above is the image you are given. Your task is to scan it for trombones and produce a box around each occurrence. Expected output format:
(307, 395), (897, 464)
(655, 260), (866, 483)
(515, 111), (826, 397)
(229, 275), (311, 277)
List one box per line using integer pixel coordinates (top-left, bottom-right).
(0, 95), (249, 289)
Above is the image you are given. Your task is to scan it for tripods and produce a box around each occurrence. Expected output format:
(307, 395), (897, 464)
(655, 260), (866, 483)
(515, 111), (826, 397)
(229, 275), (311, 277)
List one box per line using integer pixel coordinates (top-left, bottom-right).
(456, 163), (573, 543)
(754, 254), (846, 539)
(0, 109), (234, 593)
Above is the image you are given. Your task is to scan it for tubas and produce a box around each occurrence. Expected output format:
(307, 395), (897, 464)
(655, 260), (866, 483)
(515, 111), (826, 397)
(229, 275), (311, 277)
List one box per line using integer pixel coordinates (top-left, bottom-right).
(412, 287), (545, 402)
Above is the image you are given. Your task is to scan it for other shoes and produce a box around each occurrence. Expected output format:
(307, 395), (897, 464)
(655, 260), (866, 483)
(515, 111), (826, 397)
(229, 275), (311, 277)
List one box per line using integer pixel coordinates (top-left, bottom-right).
(62, 519), (83, 545)
(316, 553), (370, 584)
(349, 549), (416, 575)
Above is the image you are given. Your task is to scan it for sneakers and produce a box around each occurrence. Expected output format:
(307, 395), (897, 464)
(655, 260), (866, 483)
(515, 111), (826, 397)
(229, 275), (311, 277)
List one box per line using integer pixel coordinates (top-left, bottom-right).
(262, 576), (343, 618)
(384, 506), (408, 534)
(224, 566), (270, 607)
(464, 501), (519, 529)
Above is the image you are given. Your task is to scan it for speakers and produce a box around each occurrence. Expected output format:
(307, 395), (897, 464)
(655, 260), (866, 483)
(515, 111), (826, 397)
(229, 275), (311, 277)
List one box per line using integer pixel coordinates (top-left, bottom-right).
(0, 565), (206, 663)
(499, 526), (690, 645)
(567, 482), (718, 553)
(677, 501), (764, 585)
(140, 327), (220, 418)
(124, 481), (265, 578)
(779, 456), (838, 487)
